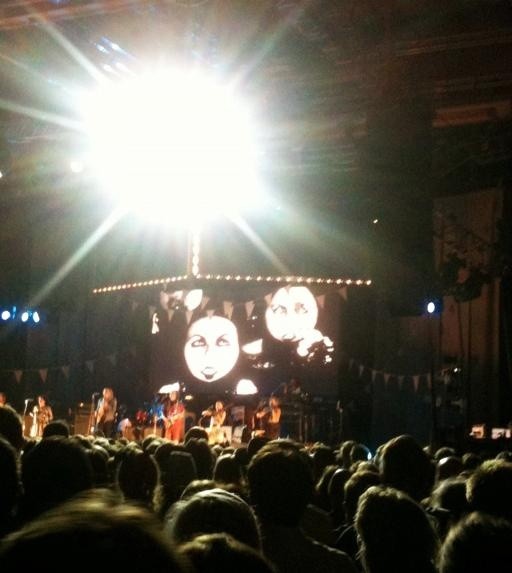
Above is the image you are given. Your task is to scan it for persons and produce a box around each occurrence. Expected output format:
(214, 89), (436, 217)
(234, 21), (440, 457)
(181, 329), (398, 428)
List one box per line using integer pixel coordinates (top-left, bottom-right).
(2, 366), (510, 573)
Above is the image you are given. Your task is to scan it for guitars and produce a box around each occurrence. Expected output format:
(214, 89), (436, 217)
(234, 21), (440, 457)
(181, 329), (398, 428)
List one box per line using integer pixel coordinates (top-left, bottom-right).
(164, 411), (187, 426)
